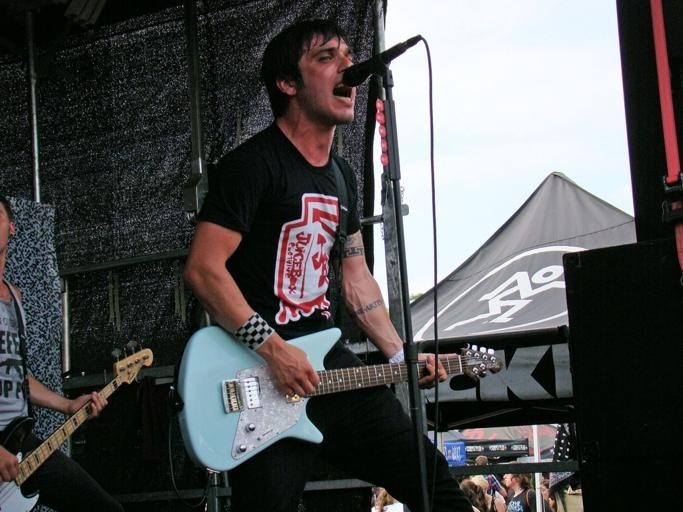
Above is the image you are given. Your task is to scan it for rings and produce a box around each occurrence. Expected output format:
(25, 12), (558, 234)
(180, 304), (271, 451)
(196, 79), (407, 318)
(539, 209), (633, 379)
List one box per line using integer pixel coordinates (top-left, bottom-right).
(289, 391), (294, 397)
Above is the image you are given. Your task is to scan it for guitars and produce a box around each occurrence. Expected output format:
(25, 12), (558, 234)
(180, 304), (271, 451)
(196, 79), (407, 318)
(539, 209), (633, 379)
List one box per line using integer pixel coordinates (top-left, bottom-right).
(173, 325), (504, 474)
(0, 343), (154, 511)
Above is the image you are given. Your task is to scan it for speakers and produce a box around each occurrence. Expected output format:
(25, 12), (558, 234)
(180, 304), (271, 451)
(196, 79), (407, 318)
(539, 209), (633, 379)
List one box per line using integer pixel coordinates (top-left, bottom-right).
(562, 235), (681, 512)
(615, 0), (682, 243)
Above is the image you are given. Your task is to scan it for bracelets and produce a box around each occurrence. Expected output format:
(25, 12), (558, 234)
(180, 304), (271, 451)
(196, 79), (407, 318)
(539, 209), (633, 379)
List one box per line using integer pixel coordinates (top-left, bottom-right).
(233, 313), (274, 350)
(389, 348), (406, 363)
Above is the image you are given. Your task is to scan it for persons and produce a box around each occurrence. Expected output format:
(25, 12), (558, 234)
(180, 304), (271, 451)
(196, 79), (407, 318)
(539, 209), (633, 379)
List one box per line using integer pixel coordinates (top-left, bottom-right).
(1, 197), (123, 512)
(181, 19), (474, 512)
(370, 456), (557, 512)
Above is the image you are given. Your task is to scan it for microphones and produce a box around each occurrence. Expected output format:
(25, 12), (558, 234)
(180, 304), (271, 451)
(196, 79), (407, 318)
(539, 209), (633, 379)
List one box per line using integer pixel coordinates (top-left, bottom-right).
(342, 34), (422, 88)
